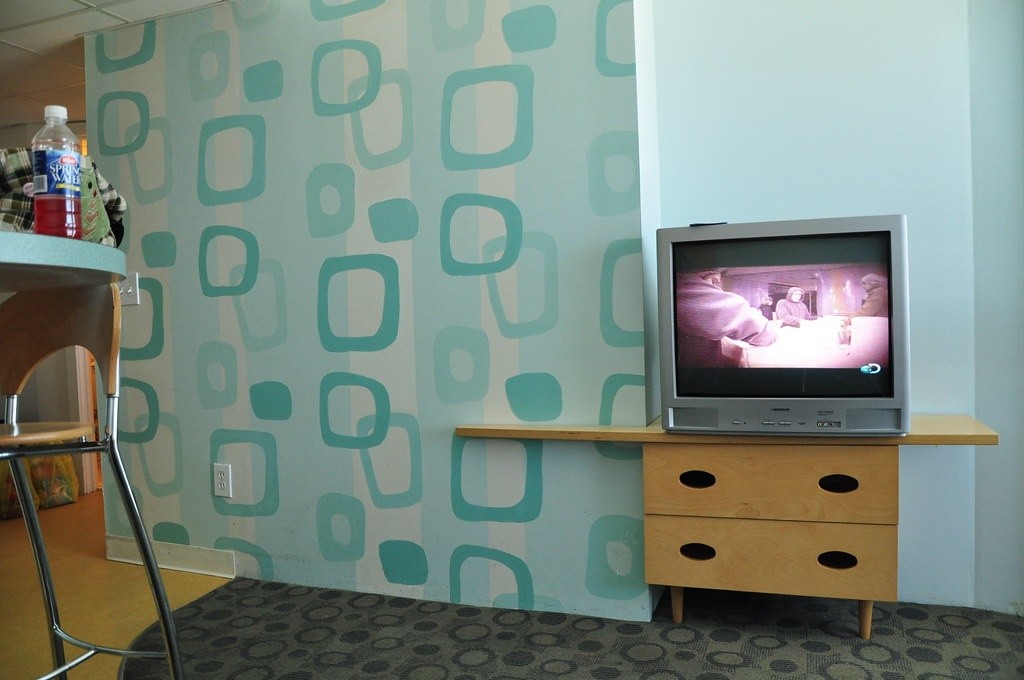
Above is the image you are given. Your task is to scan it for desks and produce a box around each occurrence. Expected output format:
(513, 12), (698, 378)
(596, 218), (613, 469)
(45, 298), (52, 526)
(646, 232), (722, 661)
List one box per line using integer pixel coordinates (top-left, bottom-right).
(0, 231), (128, 293)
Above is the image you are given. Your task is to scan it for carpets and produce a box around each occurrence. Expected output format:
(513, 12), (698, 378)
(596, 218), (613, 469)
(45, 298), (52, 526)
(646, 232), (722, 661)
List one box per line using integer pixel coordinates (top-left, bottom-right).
(120, 575), (1024, 680)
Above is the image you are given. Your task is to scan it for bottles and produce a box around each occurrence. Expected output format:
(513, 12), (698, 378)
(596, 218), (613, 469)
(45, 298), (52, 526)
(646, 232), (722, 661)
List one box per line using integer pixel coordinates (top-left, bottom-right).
(32, 105), (82, 240)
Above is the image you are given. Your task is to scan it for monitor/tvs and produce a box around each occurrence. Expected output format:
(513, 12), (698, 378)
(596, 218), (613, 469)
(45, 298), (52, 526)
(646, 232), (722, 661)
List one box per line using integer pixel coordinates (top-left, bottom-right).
(654, 214), (912, 438)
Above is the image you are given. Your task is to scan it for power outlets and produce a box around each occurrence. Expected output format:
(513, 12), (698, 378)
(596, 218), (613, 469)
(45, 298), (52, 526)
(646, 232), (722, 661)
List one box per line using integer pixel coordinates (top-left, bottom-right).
(213, 463), (233, 498)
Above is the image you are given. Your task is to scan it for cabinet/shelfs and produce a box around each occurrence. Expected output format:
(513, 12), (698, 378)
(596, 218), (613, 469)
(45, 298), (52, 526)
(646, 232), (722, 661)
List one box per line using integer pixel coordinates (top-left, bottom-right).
(460, 413), (1000, 640)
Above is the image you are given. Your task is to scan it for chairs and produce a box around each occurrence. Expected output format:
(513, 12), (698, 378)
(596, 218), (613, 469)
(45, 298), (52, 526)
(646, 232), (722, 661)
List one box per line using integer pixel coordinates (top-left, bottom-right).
(0, 282), (184, 680)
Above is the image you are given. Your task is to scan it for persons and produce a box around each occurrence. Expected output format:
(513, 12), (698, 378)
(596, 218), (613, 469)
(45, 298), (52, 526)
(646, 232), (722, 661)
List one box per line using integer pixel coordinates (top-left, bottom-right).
(851, 273), (889, 317)
(776, 286), (821, 330)
(677, 266), (784, 372)
